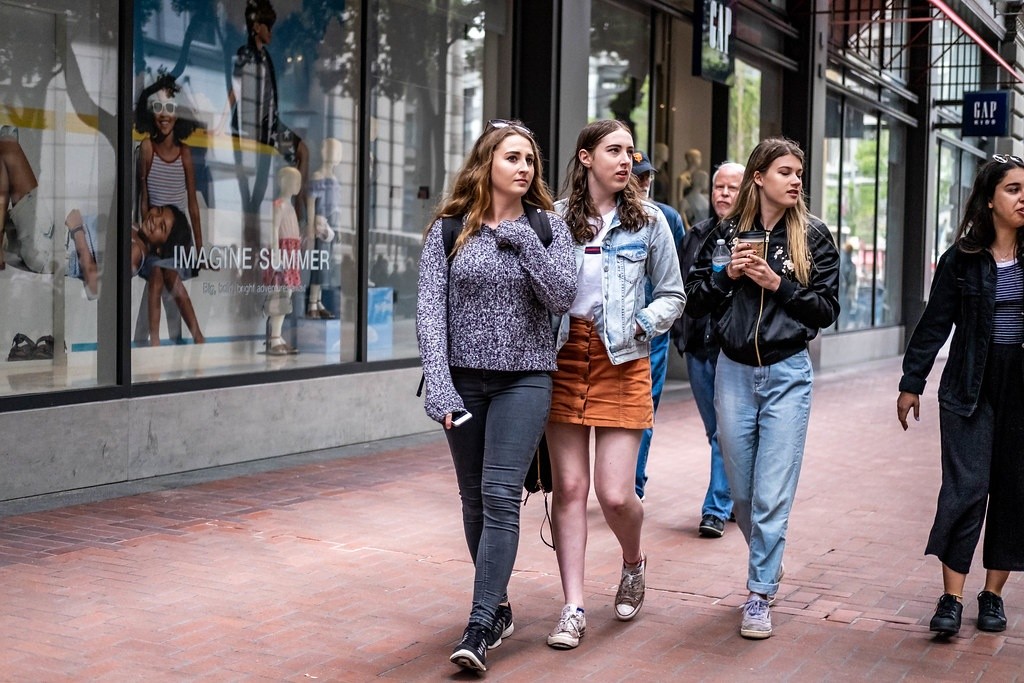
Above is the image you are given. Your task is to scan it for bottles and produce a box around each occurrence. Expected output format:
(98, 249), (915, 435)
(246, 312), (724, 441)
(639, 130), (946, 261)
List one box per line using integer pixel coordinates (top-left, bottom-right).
(712, 240), (730, 273)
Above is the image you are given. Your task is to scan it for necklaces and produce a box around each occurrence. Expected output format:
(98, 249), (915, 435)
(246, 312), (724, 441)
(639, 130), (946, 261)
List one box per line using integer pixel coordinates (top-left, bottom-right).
(992, 241), (1015, 262)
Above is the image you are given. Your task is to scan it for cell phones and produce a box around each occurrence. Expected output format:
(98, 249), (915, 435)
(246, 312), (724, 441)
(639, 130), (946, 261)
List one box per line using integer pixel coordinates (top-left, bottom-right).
(450, 410), (472, 427)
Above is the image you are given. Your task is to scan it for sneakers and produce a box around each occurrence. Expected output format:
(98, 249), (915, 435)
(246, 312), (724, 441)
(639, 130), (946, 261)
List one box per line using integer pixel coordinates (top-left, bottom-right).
(546, 604), (586, 649)
(615, 550), (646, 621)
(728, 512), (736, 521)
(699, 514), (724, 534)
(765, 562), (784, 605)
(736, 597), (775, 638)
(976, 591), (1006, 632)
(451, 626), (488, 672)
(930, 594), (963, 633)
(488, 602), (515, 649)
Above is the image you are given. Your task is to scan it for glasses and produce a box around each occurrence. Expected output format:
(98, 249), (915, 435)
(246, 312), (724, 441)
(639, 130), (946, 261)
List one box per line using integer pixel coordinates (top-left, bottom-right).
(149, 101), (178, 115)
(984, 154), (1023, 164)
(482, 119), (534, 139)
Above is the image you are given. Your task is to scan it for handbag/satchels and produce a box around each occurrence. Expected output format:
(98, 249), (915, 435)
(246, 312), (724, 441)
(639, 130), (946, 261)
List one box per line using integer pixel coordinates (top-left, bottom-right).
(522, 428), (555, 552)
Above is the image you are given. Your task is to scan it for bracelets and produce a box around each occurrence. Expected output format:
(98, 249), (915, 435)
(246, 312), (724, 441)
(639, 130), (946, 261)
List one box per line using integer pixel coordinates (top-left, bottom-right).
(727, 265), (740, 279)
(71, 225), (85, 240)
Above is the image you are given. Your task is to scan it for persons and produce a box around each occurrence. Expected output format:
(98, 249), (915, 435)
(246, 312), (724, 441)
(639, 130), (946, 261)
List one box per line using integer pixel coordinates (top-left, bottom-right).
(897, 154), (1024, 632)
(677, 148), (701, 213)
(679, 170), (709, 227)
(632, 151), (685, 503)
(0, 66), (220, 346)
(262, 166), (301, 356)
(685, 138), (840, 640)
(303, 137), (343, 320)
(416, 119), (578, 672)
(545, 119), (687, 650)
(368, 115), (379, 287)
(838, 243), (856, 331)
(654, 142), (671, 206)
(204, 0), (309, 224)
(669, 163), (746, 538)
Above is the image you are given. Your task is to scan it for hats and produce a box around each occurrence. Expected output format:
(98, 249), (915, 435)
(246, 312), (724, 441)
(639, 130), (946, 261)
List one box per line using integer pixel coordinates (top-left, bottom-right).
(632, 151), (658, 175)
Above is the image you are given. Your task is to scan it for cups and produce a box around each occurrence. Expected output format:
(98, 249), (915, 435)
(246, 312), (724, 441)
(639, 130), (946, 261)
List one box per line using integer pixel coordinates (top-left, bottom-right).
(739, 231), (764, 261)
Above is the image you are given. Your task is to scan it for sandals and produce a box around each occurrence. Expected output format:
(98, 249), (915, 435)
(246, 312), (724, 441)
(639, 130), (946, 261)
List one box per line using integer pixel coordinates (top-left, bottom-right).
(32, 334), (68, 357)
(7, 333), (37, 361)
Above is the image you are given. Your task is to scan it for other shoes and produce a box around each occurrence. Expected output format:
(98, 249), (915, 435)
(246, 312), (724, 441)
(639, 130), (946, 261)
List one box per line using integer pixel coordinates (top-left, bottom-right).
(267, 336), (299, 355)
(307, 309), (336, 320)
